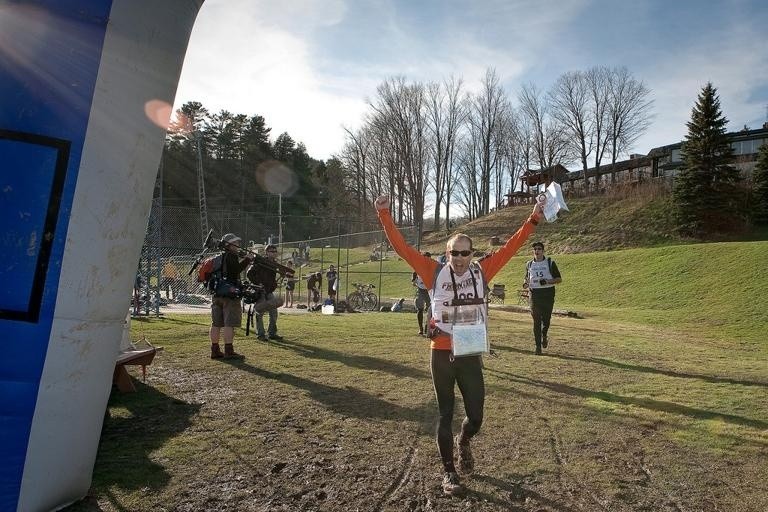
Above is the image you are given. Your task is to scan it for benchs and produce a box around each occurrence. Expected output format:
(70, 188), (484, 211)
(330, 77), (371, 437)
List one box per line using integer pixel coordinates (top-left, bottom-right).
(114, 309), (163, 393)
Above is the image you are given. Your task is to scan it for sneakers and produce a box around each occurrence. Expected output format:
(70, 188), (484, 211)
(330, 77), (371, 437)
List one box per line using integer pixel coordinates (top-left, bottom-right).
(454, 434), (474, 473)
(535, 348), (541, 355)
(442, 472), (461, 494)
(257, 336), (268, 341)
(542, 337), (547, 348)
(269, 335), (282, 340)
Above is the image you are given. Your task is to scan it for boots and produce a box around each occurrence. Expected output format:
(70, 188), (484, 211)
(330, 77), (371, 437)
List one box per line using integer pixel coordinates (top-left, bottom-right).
(212, 344), (223, 358)
(224, 344), (244, 360)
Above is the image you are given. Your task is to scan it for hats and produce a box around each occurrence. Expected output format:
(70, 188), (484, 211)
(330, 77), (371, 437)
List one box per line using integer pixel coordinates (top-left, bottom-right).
(221, 234), (242, 246)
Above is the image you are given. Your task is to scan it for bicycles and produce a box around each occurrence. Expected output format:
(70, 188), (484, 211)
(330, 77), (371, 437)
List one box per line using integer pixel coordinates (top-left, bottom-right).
(345, 281), (378, 313)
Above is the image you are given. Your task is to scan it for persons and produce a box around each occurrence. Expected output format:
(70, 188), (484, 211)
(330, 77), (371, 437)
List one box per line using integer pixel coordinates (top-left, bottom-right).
(373, 193), (545, 496)
(370, 250), (379, 261)
(160, 233), (338, 360)
(522, 241), (562, 355)
(411, 251), (431, 335)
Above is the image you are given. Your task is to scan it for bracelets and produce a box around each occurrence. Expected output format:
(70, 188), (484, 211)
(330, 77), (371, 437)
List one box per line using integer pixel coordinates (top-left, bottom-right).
(527, 217), (538, 226)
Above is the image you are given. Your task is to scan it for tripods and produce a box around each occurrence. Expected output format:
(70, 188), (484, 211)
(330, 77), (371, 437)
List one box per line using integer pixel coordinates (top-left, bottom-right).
(219, 240), (295, 279)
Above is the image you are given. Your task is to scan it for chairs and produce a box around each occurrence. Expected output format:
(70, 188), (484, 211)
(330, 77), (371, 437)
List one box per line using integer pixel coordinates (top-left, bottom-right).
(518, 290), (530, 306)
(488, 284), (505, 305)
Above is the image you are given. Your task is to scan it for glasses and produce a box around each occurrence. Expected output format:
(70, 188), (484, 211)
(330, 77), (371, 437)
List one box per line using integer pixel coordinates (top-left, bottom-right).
(448, 250), (471, 256)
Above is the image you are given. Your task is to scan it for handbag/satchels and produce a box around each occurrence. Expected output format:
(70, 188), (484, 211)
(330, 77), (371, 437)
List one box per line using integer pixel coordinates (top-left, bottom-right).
(197, 254), (224, 286)
